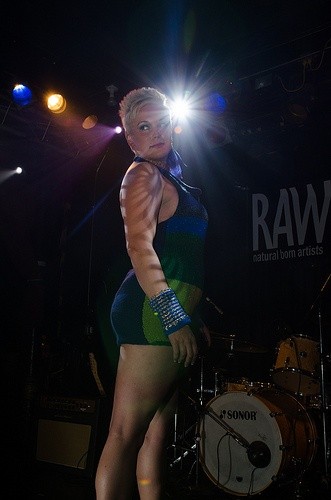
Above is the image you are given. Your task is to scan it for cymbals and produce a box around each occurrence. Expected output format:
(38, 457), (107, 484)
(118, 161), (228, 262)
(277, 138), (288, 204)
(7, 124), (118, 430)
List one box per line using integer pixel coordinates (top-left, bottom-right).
(210, 343), (268, 353)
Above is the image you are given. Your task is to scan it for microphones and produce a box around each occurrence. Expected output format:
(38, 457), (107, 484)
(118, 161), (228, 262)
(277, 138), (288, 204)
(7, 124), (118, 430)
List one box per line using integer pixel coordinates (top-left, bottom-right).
(205, 295), (223, 315)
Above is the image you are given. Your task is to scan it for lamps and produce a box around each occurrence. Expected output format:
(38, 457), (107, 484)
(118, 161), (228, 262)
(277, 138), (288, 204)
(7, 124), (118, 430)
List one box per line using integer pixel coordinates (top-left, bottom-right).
(287, 86), (320, 124)
(71, 101), (101, 130)
(40, 85), (68, 115)
(0, 74), (41, 110)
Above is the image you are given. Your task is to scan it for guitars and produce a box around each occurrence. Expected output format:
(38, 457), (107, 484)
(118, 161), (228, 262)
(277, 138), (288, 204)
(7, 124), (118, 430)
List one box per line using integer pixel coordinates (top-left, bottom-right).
(88, 352), (107, 401)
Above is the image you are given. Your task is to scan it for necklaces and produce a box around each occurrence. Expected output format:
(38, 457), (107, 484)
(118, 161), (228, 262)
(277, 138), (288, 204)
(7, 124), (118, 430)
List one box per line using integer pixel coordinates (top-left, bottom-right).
(137, 155), (167, 168)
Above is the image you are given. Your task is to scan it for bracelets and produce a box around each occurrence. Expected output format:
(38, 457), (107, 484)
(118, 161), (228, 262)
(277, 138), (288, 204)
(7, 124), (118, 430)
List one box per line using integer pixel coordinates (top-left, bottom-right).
(148, 288), (190, 335)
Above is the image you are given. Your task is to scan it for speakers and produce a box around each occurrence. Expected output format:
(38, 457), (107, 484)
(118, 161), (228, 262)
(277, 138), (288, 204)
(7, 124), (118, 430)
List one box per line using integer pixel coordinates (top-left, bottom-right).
(34, 412), (105, 471)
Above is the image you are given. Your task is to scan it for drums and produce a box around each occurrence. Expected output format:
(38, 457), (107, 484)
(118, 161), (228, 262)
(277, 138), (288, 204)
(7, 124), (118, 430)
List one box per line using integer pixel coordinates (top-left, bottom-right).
(196, 388), (318, 497)
(269, 333), (322, 397)
(226, 378), (275, 391)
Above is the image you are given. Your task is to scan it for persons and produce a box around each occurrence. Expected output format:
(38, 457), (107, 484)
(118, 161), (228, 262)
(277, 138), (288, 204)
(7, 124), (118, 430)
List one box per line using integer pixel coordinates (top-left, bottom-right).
(95, 86), (211, 500)
